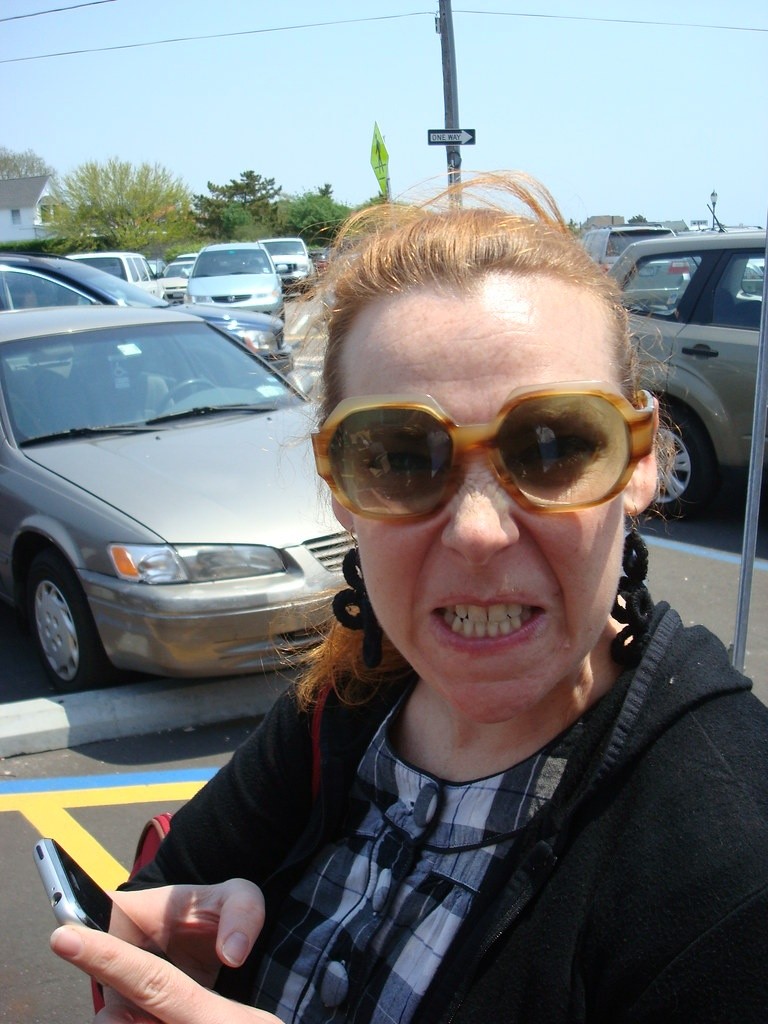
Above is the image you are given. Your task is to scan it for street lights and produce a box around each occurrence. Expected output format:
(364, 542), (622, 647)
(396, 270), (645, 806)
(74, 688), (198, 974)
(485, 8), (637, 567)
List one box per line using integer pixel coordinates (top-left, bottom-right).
(710, 189), (717, 228)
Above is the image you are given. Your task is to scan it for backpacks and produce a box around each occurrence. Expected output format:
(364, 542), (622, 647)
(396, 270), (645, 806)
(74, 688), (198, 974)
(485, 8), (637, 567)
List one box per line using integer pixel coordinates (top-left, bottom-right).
(89, 659), (363, 1024)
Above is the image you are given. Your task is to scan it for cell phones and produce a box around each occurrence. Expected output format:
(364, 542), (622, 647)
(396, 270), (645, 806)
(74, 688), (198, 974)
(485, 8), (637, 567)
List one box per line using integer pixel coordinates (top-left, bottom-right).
(33, 839), (178, 1024)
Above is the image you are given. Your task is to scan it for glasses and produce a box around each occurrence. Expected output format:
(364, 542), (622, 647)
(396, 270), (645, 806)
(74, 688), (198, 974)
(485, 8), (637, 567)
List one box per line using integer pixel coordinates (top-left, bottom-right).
(310, 378), (655, 521)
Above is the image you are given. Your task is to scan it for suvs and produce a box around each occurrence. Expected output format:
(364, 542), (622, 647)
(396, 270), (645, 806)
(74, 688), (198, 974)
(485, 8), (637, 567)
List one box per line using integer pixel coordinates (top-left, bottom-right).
(606, 231), (768, 520)
(0, 251), (294, 378)
(580, 223), (765, 311)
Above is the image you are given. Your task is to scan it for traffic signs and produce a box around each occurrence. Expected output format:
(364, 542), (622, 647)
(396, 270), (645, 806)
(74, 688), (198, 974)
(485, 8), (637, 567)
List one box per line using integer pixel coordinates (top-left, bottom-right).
(427, 128), (476, 145)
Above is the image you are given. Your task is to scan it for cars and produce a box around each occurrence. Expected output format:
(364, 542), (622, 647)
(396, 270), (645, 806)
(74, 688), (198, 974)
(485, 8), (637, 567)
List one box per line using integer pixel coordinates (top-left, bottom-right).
(0, 305), (359, 694)
(65, 252), (199, 306)
(181, 242), (288, 324)
(258, 238), (363, 301)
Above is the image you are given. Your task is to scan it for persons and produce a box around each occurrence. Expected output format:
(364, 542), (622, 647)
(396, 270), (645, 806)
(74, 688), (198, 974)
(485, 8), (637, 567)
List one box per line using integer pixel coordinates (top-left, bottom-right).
(50, 168), (768, 1024)
